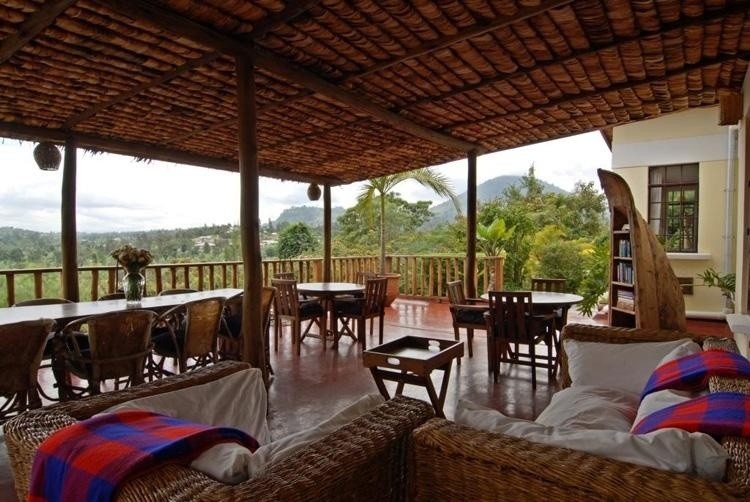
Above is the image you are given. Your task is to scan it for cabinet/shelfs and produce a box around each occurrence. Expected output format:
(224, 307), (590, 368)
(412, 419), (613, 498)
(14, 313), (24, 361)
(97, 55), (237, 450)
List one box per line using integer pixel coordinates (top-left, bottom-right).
(597, 168), (687, 329)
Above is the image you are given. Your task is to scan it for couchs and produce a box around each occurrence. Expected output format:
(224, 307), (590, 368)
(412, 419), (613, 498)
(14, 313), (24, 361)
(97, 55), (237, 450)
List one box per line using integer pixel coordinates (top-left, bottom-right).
(3, 359), (436, 502)
(413, 322), (750, 502)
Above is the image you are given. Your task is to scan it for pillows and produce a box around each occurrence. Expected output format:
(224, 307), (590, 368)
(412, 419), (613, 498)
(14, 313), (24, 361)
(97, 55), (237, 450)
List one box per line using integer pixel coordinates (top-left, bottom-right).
(96, 368), (271, 444)
(453, 398), (692, 479)
(248, 392), (385, 484)
(92, 408), (250, 482)
(630, 343), (705, 437)
(561, 338), (691, 390)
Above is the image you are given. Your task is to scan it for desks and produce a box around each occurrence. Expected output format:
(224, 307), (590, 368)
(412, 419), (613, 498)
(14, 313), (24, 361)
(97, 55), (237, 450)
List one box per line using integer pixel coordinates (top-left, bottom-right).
(290, 282), (367, 344)
(0, 288), (245, 338)
(480, 291), (585, 384)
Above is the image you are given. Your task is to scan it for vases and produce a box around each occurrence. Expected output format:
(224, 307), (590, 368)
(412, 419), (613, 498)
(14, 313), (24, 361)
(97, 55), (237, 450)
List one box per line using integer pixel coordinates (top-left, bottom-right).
(122, 268), (145, 303)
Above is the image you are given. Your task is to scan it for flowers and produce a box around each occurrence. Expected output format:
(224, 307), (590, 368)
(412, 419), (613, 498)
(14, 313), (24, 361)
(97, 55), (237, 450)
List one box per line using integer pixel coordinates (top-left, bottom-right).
(111, 245), (155, 300)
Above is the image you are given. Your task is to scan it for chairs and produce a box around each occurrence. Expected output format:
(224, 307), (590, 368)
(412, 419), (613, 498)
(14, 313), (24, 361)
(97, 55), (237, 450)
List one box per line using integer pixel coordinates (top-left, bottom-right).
(447, 280), (508, 365)
(149, 297), (226, 378)
(488, 291), (556, 392)
(334, 279), (388, 354)
(274, 273), (322, 342)
(336, 272), (375, 337)
(273, 279), (325, 359)
(55, 309), (159, 401)
(1, 319), (55, 427)
(218, 286), (276, 377)
(515, 278), (568, 370)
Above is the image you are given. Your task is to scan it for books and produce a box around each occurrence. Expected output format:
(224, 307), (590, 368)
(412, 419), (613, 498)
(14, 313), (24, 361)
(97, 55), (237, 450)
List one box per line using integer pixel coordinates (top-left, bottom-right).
(617, 262), (634, 284)
(619, 239), (631, 257)
(617, 290), (634, 311)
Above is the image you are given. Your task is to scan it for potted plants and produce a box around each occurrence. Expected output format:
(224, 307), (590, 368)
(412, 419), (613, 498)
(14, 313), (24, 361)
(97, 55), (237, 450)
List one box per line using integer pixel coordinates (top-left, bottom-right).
(356, 168), (465, 308)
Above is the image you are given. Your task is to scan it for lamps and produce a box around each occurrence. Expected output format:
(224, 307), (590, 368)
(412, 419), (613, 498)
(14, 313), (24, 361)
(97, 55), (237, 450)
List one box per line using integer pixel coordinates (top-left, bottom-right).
(33, 141), (60, 172)
(307, 184), (320, 201)
